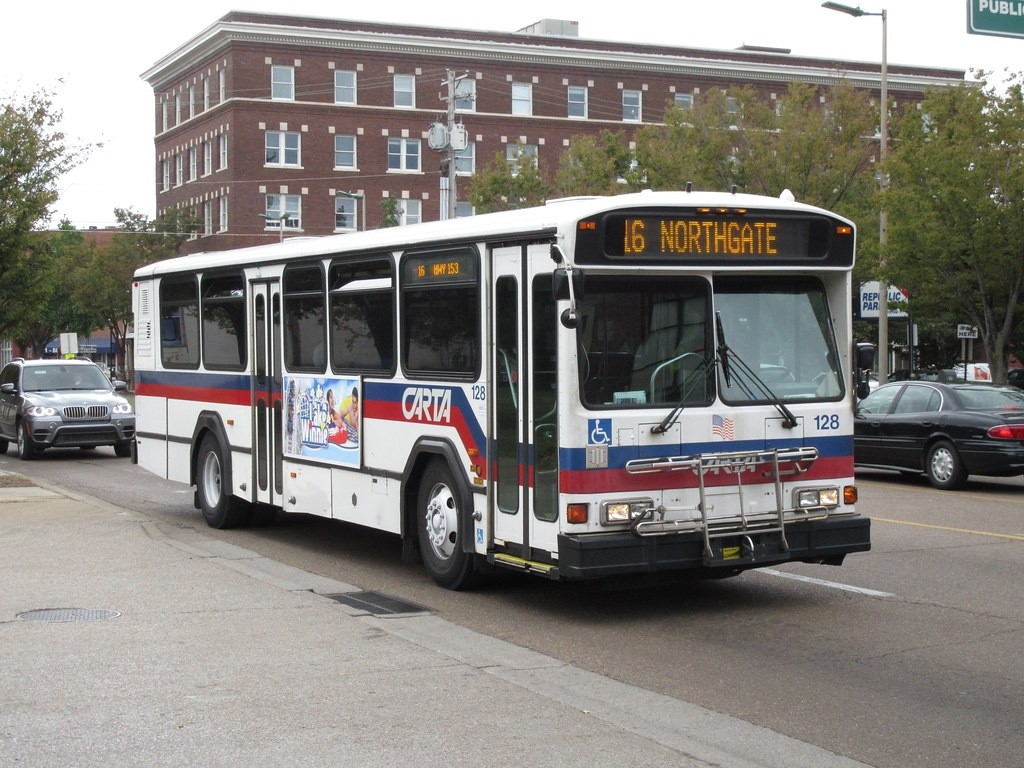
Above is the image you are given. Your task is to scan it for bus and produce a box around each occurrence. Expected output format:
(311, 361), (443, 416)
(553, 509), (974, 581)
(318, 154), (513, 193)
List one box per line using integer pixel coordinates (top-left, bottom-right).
(125, 185), (874, 594)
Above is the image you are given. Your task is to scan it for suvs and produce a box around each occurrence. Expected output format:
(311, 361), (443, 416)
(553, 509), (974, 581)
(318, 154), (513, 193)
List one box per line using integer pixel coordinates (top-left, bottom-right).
(1, 354), (136, 461)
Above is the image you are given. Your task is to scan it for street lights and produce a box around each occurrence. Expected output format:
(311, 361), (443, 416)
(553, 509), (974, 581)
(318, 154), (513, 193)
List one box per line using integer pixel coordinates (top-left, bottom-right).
(337, 189), (367, 232)
(822, 1), (890, 393)
(257, 210), (284, 244)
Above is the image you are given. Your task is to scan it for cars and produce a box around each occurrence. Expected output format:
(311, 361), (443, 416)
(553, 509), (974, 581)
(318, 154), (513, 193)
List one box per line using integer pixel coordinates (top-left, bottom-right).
(852, 381), (1023, 490)
